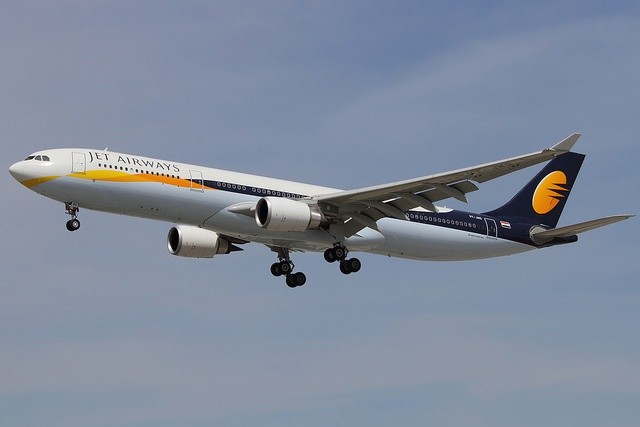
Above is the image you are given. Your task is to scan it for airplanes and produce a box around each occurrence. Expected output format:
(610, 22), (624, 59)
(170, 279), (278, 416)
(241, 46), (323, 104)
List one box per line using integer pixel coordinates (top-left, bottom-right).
(8, 133), (638, 288)
